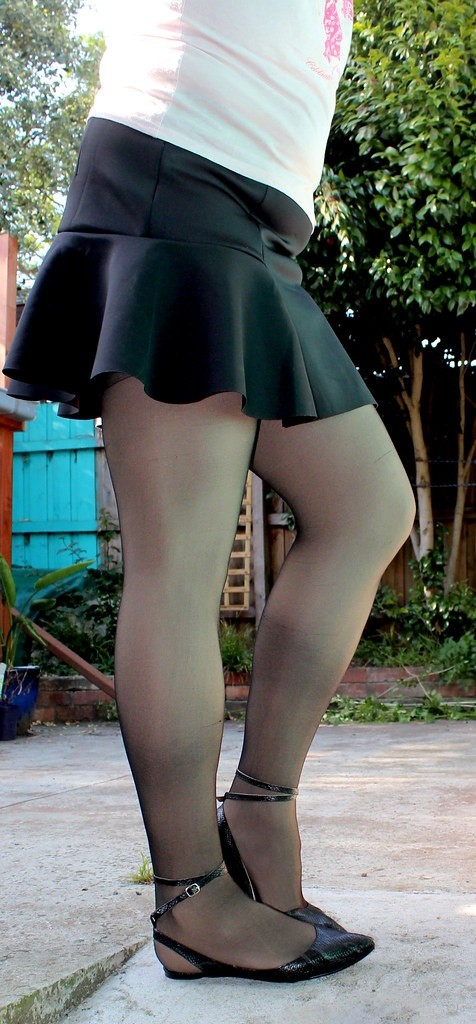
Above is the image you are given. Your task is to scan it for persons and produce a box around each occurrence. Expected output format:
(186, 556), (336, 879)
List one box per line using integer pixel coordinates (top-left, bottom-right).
(3, 0), (419, 984)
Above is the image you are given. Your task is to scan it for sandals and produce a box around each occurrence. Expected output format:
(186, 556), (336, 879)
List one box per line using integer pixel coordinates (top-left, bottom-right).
(217, 771), (348, 932)
(150, 861), (375, 983)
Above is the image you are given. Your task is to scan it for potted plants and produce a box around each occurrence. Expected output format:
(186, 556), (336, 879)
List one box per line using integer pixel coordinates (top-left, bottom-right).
(0, 554), (96, 741)
